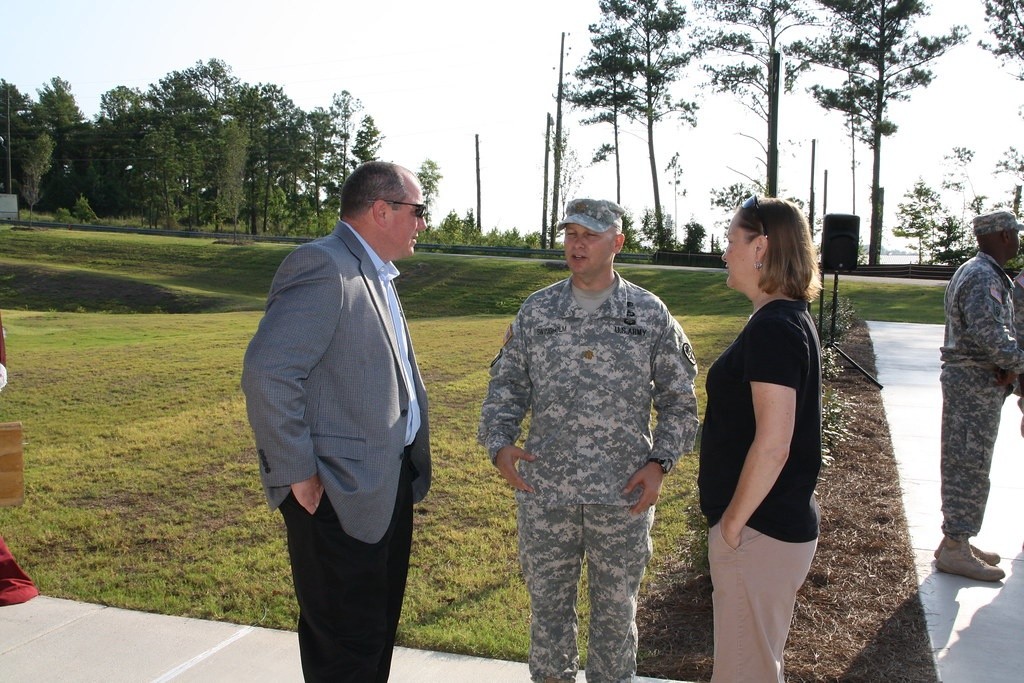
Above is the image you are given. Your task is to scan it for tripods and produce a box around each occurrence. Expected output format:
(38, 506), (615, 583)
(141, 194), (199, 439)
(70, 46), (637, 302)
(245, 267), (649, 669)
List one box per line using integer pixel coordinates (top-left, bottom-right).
(823, 268), (884, 389)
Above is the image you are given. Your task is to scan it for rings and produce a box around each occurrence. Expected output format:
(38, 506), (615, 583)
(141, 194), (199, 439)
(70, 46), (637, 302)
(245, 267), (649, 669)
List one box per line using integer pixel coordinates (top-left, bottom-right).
(650, 503), (654, 506)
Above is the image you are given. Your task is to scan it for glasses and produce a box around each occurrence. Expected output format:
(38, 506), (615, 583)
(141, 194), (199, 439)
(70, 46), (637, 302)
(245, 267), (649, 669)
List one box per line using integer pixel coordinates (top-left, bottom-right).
(384, 199), (428, 219)
(742, 195), (765, 235)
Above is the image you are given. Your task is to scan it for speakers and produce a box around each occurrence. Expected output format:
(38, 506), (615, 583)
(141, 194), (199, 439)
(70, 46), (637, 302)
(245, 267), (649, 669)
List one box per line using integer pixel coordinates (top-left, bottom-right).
(820, 215), (860, 271)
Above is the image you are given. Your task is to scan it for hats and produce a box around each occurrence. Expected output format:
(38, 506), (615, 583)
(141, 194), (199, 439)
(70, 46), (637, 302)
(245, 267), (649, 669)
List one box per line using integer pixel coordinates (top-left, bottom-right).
(972, 211), (1024, 236)
(557, 198), (627, 233)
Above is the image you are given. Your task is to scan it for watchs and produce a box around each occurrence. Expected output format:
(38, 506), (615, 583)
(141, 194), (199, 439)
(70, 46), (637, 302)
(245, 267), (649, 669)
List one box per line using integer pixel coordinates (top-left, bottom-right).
(647, 458), (673, 473)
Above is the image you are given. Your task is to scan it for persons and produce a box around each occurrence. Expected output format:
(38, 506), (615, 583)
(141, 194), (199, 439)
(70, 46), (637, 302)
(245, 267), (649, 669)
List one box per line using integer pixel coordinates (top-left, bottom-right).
(697, 191), (822, 683)
(476, 198), (698, 683)
(935, 210), (1024, 581)
(242, 161), (433, 683)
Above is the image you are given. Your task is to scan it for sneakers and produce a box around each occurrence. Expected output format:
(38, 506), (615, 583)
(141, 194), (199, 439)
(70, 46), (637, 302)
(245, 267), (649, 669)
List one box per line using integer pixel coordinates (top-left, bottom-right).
(934, 533), (1006, 581)
(932, 534), (1001, 565)
